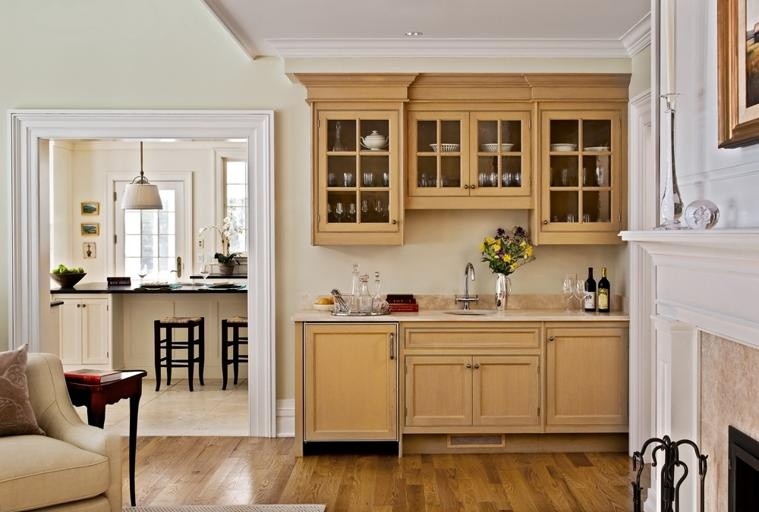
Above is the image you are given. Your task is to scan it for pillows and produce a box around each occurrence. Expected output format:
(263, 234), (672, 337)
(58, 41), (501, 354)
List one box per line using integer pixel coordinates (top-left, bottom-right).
(0, 344), (46, 435)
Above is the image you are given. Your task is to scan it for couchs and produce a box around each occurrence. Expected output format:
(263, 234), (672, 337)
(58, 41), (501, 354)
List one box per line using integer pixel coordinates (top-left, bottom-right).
(0, 353), (123, 512)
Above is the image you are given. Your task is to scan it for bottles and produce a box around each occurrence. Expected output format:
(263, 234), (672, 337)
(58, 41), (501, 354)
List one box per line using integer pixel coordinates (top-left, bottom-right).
(418, 173), (451, 186)
(358, 276), (372, 314)
(350, 265), (359, 313)
(597, 266), (610, 312)
(371, 273), (388, 315)
(331, 122), (348, 151)
(584, 267), (597, 311)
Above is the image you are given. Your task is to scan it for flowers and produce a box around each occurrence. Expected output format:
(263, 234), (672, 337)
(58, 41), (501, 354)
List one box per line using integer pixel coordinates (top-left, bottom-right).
(479, 226), (536, 276)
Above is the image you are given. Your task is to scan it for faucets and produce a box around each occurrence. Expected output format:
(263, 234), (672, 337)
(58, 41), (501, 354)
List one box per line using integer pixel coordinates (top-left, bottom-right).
(197, 224), (226, 256)
(464, 262), (476, 299)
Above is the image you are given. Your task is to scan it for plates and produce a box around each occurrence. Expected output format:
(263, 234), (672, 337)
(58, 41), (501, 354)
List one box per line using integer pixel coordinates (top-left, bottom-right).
(584, 147), (608, 151)
(313, 303), (335, 310)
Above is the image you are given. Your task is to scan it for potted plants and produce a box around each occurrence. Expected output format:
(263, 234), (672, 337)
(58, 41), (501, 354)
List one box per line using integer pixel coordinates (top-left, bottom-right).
(215, 253), (240, 274)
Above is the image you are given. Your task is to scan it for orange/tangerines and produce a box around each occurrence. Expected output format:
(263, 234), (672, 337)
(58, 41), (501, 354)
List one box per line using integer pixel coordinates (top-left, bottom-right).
(318, 296), (334, 306)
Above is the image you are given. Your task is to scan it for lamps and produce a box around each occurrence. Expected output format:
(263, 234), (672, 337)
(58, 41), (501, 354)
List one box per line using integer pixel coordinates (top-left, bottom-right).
(122, 141), (162, 209)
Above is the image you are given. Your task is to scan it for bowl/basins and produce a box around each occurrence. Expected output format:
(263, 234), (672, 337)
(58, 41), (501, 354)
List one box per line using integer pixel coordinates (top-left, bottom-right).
(49, 273), (87, 288)
(552, 143), (577, 151)
(552, 214), (591, 223)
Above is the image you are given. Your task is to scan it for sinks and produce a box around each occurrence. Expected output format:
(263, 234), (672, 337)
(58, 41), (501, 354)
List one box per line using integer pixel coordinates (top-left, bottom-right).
(443, 309), (499, 317)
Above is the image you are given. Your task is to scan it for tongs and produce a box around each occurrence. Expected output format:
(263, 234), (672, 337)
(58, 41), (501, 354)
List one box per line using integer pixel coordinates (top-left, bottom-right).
(331, 289), (348, 310)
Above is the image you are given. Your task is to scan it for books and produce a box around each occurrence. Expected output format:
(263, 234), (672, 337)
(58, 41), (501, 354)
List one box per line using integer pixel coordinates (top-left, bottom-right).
(64, 368), (122, 384)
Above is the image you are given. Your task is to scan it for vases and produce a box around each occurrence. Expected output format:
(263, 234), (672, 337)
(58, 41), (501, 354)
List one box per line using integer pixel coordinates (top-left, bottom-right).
(495, 273), (512, 311)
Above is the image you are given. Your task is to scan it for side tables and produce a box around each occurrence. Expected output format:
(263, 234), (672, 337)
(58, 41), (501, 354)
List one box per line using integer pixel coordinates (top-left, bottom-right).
(67, 370), (147, 506)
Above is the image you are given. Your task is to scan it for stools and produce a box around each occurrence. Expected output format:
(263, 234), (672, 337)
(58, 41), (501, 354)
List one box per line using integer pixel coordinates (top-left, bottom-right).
(222, 316), (248, 390)
(155, 317), (204, 392)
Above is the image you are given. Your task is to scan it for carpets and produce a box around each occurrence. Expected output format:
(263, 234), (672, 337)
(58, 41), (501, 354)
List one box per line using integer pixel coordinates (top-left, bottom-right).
(123, 504), (326, 512)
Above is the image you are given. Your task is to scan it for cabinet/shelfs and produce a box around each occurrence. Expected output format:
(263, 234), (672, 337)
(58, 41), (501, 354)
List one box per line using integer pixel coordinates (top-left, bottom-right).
(294, 73), (420, 247)
(295, 321), (402, 460)
(522, 73), (632, 246)
(404, 74), (534, 209)
(59, 293), (123, 370)
(401, 322), (544, 435)
(545, 322), (629, 433)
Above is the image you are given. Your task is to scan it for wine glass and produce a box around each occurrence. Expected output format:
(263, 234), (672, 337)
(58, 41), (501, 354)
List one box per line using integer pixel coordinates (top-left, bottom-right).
(199, 264), (211, 290)
(138, 263), (147, 285)
(573, 281), (588, 316)
(560, 278), (575, 315)
(328, 200), (389, 222)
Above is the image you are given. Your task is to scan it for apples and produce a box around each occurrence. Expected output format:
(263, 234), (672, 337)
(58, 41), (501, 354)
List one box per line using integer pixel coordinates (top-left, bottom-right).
(51, 264), (84, 274)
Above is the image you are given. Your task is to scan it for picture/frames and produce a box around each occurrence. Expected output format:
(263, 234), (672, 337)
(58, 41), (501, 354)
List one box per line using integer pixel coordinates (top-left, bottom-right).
(718, 0), (759, 148)
(81, 223), (99, 237)
(83, 242), (96, 259)
(80, 201), (99, 216)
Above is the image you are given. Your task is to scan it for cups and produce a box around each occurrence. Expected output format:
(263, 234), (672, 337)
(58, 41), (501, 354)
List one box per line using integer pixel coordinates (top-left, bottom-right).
(333, 295), (353, 315)
(328, 170), (388, 187)
(550, 167), (589, 186)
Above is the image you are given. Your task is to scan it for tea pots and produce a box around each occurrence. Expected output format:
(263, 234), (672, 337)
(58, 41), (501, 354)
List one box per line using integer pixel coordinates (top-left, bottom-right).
(478, 170), (520, 186)
(360, 130), (389, 150)
